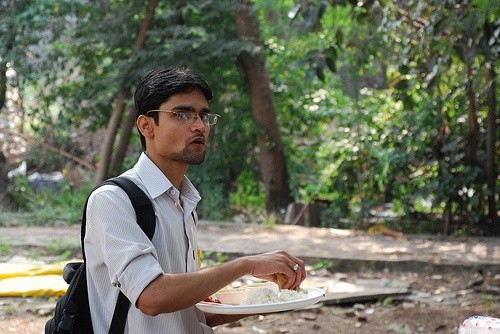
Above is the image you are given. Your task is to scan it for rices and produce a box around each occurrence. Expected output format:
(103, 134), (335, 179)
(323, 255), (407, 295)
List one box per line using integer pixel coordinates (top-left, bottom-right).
(238, 287), (308, 306)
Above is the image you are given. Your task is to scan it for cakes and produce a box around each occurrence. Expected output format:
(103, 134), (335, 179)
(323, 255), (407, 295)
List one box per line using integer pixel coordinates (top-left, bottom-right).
(463, 315), (500, 332)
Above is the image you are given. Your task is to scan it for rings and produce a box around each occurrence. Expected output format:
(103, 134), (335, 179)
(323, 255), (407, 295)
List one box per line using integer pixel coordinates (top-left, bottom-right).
(293, 263), (298, 271)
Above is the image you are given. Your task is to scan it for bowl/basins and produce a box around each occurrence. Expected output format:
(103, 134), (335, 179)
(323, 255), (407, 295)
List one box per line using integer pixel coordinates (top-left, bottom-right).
(242, 283), (279, 301)
(216, 288), (250, 305)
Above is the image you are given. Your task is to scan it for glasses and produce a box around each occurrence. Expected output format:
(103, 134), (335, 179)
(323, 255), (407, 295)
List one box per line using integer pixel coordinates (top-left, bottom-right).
(146, 110), (221, 125)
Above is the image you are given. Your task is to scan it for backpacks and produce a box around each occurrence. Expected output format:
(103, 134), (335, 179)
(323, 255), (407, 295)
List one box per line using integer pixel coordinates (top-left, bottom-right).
(44, 177), (157, 334)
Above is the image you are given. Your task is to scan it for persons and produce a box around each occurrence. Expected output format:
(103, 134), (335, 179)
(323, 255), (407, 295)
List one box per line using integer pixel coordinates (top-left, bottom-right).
(81, 66), (306, 334)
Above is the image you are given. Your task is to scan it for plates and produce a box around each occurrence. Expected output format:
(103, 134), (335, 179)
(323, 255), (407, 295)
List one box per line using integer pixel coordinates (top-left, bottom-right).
(194, 287), (326, 315)
